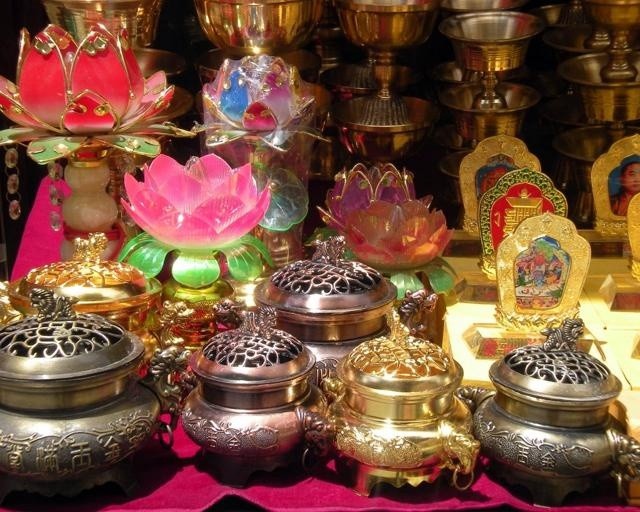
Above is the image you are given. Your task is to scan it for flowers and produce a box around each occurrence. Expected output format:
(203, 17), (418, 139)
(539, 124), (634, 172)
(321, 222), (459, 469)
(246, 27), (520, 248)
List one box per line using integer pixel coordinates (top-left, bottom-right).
(201, 54), (326, 155)
(116, 152), (278, 291)
(306, 158), (463, 299)
(0, 21), (198, 167)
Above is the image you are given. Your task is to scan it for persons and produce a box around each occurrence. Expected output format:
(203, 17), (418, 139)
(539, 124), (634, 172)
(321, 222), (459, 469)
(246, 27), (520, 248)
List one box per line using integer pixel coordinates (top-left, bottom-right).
(602, 162), (639, 216)
